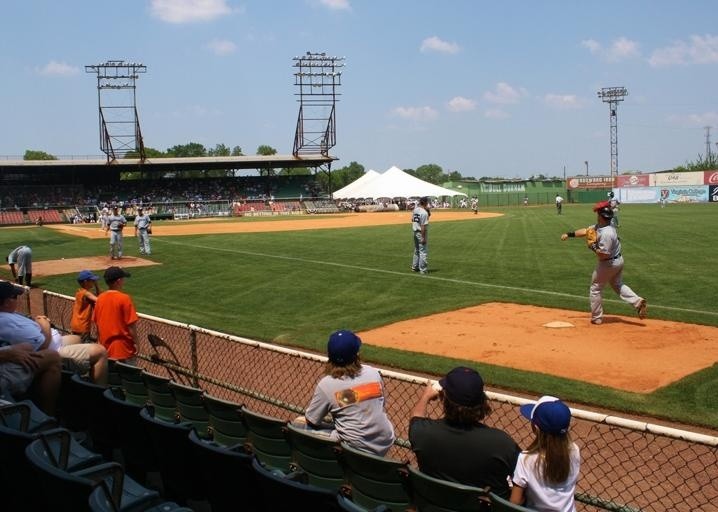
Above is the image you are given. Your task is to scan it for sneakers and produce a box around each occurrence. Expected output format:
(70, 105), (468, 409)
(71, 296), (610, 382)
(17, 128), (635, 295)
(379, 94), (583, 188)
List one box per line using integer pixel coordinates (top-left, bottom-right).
(636, 300), (650, 322)
(590, 318), (603, 327)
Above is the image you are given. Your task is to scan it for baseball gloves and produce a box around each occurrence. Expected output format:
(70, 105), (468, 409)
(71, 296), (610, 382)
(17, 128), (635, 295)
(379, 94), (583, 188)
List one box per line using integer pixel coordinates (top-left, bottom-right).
(585, 227), (597, 248)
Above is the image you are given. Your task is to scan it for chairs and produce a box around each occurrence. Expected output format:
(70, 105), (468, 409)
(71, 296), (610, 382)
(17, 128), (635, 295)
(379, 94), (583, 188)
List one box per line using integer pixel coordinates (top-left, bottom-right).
(0, 356), (543, 512)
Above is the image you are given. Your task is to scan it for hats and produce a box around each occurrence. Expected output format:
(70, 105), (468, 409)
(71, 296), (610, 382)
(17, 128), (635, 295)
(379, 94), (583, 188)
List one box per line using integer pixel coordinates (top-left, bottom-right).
(103, 266), (132, 285)
(326, 330), (361, 367)
(438, 365), (486, 407)
(0, 280), (25, 304)
(593, 200), (614, 220)
(518, 394), (572, 436)
(77, 270), (101, 282)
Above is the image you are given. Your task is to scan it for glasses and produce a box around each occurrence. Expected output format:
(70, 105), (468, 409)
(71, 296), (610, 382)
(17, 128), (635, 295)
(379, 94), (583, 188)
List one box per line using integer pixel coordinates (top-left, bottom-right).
(9, 294), (18, 300)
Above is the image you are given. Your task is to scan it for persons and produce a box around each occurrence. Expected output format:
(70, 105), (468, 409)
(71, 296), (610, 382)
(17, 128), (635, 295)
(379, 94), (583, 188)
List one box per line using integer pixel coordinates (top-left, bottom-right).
(337, 195), (415, 212)
(411, 197), (431, 275)
(509, 396), (580, 512)
(554, 194), (564, 213)
(426, 195), (450, 209)
(523, 194), (529, 207)
(0, 186), (108, 225)
(134, 206), (152, 258)
(294, 330), (394, 458)
(91, 266), (140, 366)
(561, 207), (647, 325)
(70, 270), (100, 373)
(6, 246), (32, 288)
(168, 181), (277, 215)
(105, 206), (128, 259)
(459, 195), (470, 209)
(0, 281), (107, 386)
(0, 338), (62, 417)
(298, 177), (323, 204)
(407, 367), (524, 503)
(607, 192), (620, 228)
(108, 184), (167, 214)
(470, 194), (479, 214)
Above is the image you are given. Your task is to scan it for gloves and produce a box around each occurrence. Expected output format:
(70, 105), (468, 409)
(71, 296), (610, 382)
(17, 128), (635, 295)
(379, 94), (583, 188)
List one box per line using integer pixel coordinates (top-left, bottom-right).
(585, 225), (602, 251)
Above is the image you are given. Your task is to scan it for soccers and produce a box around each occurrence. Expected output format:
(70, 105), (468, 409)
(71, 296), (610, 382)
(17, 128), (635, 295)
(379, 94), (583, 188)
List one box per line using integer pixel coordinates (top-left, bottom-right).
(118, 222), (123, 229)
(147, 228), (151, 233)
